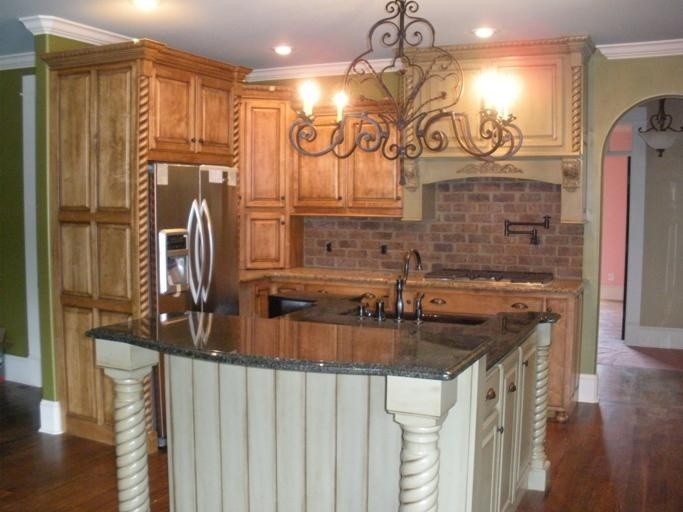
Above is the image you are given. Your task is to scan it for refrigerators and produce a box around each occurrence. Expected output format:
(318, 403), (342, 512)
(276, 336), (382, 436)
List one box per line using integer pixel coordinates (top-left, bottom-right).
(148, 161), (239, 448)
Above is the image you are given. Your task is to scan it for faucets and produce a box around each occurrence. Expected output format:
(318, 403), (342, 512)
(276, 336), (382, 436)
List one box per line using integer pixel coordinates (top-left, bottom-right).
(396, 249), (423, 320)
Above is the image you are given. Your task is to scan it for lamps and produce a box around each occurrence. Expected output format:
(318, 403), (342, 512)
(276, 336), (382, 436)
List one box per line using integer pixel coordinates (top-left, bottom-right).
(288, 1), (523, 185)
(639, 99), (683, 156)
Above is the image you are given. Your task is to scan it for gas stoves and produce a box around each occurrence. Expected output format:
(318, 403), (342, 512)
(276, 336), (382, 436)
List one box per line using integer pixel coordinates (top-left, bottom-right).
(423, 268), (554, 286)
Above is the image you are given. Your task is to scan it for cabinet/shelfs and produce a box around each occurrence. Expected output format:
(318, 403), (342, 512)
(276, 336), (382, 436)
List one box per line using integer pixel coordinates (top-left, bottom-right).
(292, 100), (403, 218)
(242, 84), (303, 271)
(475, 334), (534, 511)
(393, 36), (600, 159)
(39, 38), (254, 457)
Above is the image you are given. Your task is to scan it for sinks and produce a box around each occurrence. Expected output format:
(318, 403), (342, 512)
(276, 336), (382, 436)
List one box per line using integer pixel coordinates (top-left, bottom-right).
(348, 306), (487, 326)
